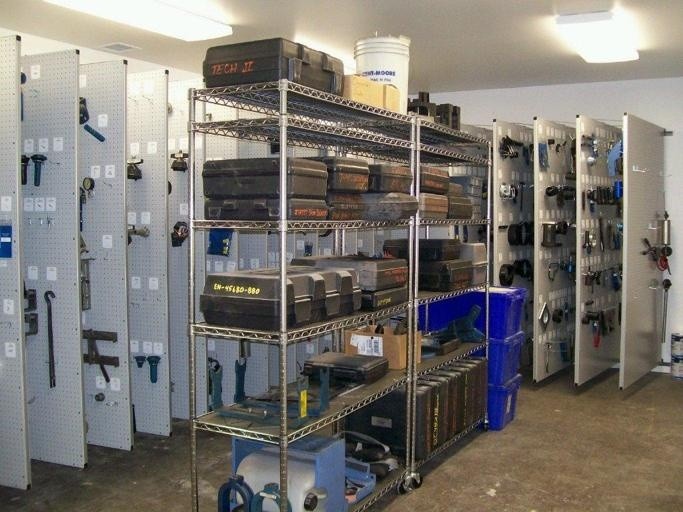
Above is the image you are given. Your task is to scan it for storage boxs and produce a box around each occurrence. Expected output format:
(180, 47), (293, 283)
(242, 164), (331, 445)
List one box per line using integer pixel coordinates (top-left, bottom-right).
(290, 256), (408, 292)
(199, 265), (363, 331)
(362, 286), (410, 312)
(448, 196), (473, 218)
(419, 259), (473, 292)
(417, 287), (527, 339)
(448, 183), (463, 195)
(419, 192), (448, 219)
(302, 351), (388, 384)
(201, 157), (329, 199)
(205, 197), (329, 222)
(416, 379), (440, 450)
(459, 359), (484, 418)
(201, 37), (345, 97)
(489, 374), (522, 431)
(428, 369), (462, 437)
(344, 385), (433, 460)
(306, 156), (370, 193)
(466, 356), (488, 415)
(414, 166), (450, 195)
(366, 192), (419, 221)
(439, 366), (471, 429)
(328, 190), (366, 220)
(472, 331), (526, 384)
(370, 163), (413, 195)
(450, 361), (479, 423)
(419, 375), (450, 444)
(383, 238), (461, 260)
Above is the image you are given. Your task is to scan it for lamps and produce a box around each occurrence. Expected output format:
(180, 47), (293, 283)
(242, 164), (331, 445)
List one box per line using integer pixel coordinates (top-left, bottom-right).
(43, 0), (234, 44)
(554, 10), (639, 65)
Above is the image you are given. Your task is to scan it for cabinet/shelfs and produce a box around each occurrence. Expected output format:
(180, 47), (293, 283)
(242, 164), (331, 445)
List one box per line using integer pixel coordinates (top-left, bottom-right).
(187, 80), (416, 510)
(410, 115), (493, 490)
